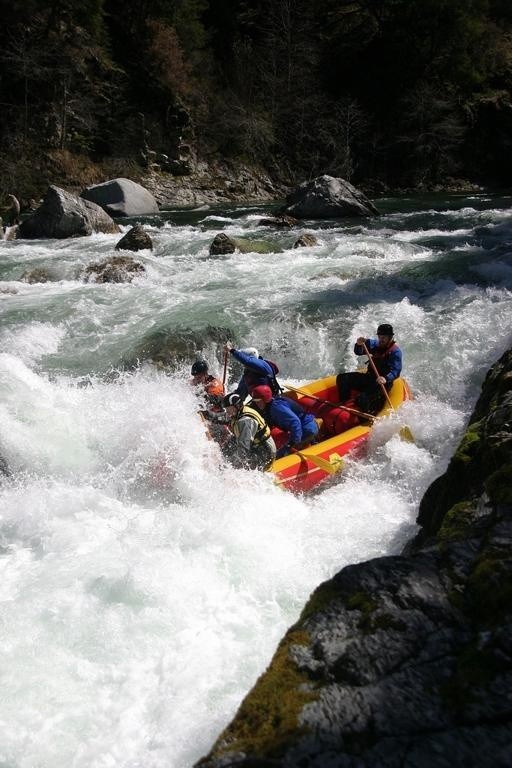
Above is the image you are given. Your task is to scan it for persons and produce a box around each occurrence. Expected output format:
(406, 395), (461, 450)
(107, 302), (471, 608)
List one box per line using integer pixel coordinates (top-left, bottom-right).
(222, 393), (277, 476)
(336, 323), (402, 415)
(189, 361), (224, 410)
(249, 384), (319, 458)
(223, 341), (278, 429)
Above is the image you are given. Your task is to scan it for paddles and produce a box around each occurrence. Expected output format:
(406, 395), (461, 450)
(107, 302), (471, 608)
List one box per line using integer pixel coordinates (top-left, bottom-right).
(289, 447), (333, 474)
(362, 342), (415, 443)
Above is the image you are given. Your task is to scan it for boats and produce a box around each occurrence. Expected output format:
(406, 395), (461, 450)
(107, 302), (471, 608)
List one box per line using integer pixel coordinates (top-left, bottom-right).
(141, 363), (415, 519)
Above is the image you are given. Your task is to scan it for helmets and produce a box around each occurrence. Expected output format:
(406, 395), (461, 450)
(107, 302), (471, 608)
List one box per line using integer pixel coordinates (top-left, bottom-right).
(252, 385), (272, 404)
(221, 393), (243, 409)
(191, 361), (208, 375)
(376, 324), (394, 335)
(239, 348), (259, 359)
